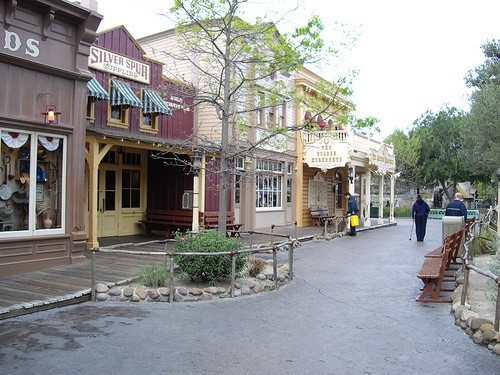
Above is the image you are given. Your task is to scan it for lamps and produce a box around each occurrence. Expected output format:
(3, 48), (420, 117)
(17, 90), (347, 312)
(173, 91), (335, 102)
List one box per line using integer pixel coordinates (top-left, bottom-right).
(191, 149), (203, 176)
(36, 92), (62, 127)
(348, 167), (354, 185)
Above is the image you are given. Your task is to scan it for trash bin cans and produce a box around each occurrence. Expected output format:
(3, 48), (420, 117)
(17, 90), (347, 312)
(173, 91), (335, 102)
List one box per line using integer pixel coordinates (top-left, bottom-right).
(442, 215), (465, 258)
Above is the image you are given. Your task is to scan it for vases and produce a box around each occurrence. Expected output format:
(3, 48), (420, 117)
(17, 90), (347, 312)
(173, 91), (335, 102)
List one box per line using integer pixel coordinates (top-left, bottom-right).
(43, 213), (52, 229)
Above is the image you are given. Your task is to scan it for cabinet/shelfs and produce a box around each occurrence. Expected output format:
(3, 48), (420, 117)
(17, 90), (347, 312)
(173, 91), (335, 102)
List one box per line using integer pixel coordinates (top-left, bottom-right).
(15, 159), (50, 183)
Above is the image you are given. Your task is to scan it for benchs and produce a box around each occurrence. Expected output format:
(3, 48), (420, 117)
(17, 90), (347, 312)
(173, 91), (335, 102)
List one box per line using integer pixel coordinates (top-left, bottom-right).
(205, 211), (245, 238)
(138, 208), (204, 239)
(415, 216), (476, 303)
(308, 207), (336, 226)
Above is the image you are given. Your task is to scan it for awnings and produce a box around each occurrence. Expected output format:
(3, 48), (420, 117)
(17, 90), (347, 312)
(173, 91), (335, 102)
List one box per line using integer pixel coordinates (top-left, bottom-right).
(110, 80), (143, 106)
(87, 76), (109, 100)
(142, 89), (170, 116)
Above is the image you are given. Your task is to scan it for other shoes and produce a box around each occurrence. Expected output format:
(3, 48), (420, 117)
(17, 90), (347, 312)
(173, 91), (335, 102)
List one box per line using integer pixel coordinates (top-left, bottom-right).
(417, 238), (424, 241)
(348, 232), (356, 236)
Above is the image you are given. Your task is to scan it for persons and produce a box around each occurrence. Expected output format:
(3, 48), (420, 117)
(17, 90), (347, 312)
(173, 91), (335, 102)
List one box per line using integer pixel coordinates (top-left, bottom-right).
(445, 193), (467, 223)
(344, 191), (360, 236)
(411, 195), (430, 241)
